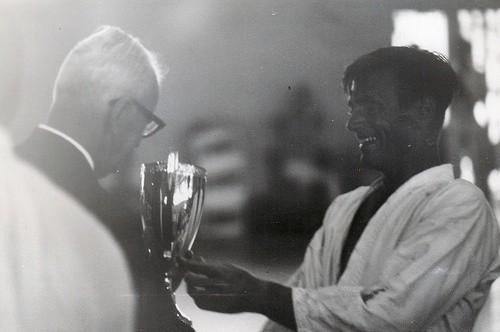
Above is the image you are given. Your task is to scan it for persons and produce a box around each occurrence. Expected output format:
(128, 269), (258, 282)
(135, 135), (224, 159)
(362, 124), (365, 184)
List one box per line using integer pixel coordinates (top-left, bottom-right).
(447, 38), (493, 203)
(185, 46), (500, 332)
(12, 26), (194, 331)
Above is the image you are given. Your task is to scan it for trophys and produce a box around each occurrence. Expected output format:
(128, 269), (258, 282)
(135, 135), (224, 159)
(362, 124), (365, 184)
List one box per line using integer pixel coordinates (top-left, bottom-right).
(139, 162), (208, 325)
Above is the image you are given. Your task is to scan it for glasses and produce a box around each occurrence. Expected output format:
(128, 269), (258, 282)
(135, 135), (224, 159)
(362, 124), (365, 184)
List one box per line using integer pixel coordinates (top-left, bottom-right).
(109, 96), (166, 138)
